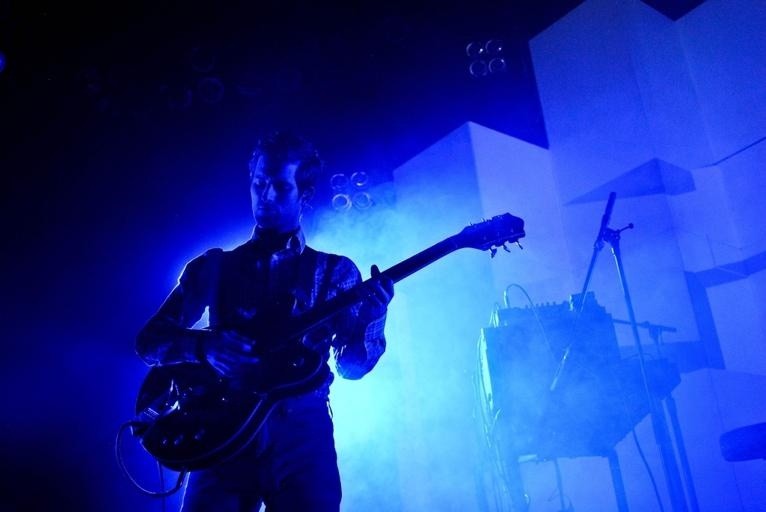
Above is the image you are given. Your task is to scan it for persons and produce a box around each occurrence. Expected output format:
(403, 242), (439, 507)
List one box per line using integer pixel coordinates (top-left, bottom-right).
(133, 129), (395, 512)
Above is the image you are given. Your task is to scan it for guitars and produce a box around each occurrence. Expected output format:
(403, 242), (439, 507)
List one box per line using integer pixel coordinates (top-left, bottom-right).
(134, 213), (525, 470)
